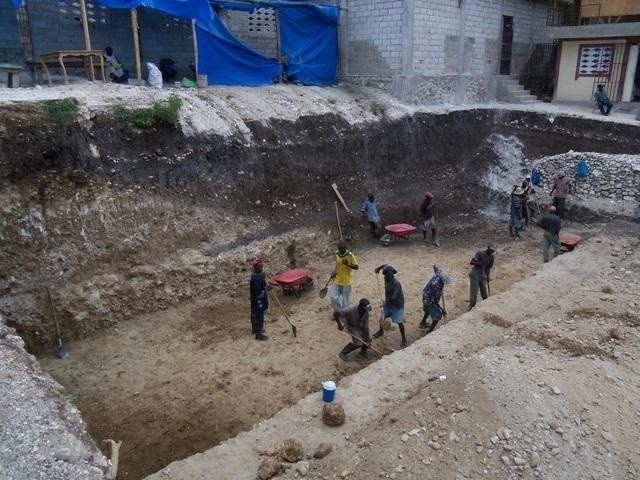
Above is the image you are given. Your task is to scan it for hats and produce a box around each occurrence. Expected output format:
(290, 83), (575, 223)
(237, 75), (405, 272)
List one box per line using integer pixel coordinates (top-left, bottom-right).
(252, 258), (264, 265)
(425, 192), (433, 197)
(548, 206), (556, 213)
(359, 299), (372, 310)
(383, 266), (397, 274)
(439, 271), (451, 284)
(514, 187), (524, 195)
(489, 243), (497, 250)
(367, 192), (375, 197)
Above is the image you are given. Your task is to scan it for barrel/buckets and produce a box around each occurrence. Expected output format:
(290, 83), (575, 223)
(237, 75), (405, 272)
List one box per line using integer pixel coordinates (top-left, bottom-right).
(196, 74), (208, 88)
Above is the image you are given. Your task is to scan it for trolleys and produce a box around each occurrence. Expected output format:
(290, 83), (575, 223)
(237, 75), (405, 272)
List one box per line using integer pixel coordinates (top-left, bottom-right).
(558, 232), (584, 253)
(384, 223), (417, 241)
(267, 268), (310, 300)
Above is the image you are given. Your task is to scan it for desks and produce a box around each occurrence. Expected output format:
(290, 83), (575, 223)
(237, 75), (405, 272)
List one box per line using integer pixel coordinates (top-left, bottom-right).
(39, 48), (108, 85)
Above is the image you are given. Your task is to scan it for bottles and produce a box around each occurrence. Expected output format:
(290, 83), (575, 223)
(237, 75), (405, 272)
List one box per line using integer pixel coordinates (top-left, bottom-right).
(174, 81), (181, 89)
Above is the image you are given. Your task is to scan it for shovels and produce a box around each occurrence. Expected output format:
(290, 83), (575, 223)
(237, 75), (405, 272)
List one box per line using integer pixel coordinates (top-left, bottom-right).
(376, 272), (392, 331)
(343, 328), (395, 358)
(319, 277), (332, 298)
(47, 287), (69, 359)
(268, 284), (297, 337)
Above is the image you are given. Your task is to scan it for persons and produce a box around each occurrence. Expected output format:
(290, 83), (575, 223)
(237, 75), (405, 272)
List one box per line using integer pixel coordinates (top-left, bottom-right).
(250, 259), (273, 341)
(329, 241), (359, 320)
(549, 170), (575, 222)
(594, 84), (612, 116)
(333, 298), (373, 361)
(102, 46), (129, 83)
(360, 192), (382, 239)
(419, 191), (441, 247)
(372, 263), (408, 349)
(467, 243), (497, 311)
(508, 174), (536, 240)
(536, 206), (561, 262)
(420, 264), (451, 334)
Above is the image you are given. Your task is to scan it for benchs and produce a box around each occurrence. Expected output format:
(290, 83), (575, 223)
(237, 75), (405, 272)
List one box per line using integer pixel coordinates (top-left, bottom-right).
(26, 61), (111, 84)
(0, 67), (24, 87)
